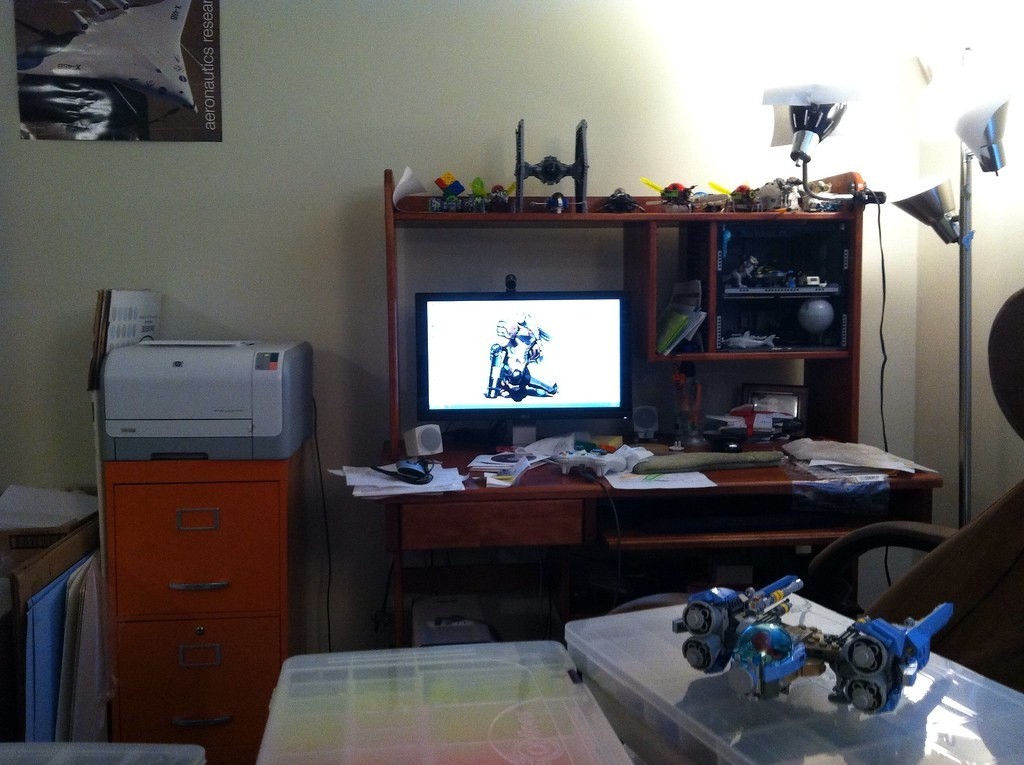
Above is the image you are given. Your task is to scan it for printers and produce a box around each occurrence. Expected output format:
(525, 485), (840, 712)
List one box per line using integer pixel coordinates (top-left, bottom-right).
(98, 339), (314, 461)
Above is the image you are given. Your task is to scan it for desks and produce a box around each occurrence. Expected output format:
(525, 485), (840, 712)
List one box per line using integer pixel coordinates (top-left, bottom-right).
(382, 438), (946, 646)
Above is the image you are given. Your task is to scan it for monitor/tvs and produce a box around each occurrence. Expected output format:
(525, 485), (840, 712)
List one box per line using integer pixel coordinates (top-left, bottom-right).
(415, 291), (631, 446)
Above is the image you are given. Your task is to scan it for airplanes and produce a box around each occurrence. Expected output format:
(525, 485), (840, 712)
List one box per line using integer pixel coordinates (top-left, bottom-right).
(16, 0), (199, 114)
(528, 192), (586, 214)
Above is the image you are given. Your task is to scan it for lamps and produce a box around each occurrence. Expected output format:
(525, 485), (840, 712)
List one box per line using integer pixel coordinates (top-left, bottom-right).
(786, 40), (1011, 531)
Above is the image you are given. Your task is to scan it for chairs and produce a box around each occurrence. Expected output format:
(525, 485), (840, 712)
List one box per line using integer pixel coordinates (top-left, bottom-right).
(803, 287), (1024, 696)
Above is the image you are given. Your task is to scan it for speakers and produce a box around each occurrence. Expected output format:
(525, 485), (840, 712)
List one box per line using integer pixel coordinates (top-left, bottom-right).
(634, 406), (659, 441)
(404, 425), (444, 464)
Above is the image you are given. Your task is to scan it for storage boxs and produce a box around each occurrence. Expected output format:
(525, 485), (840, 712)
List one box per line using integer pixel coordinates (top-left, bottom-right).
(253, 638), (637, 765)
(563, 591), (1024, 765)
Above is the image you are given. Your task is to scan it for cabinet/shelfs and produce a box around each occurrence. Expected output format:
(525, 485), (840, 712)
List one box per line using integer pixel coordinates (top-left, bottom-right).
(382, 162), (869, 440)
(99, 453), (304, 750)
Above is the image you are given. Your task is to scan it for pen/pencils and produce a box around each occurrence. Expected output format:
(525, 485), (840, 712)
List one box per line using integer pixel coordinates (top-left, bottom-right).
(472, 475), (512, 481)
